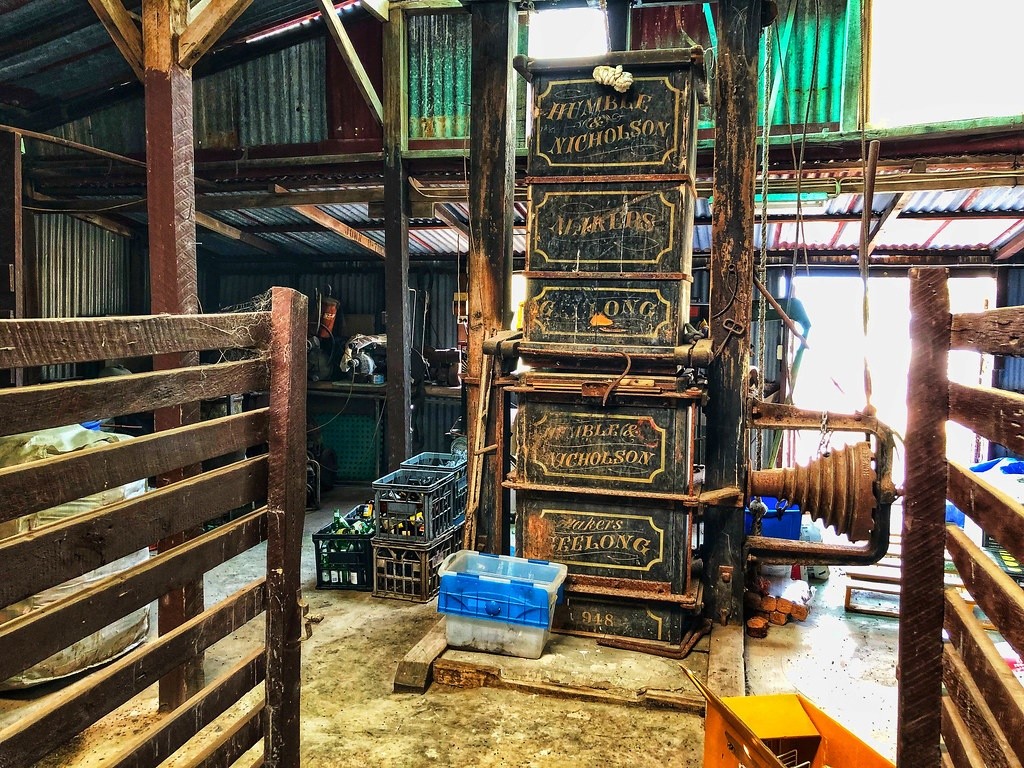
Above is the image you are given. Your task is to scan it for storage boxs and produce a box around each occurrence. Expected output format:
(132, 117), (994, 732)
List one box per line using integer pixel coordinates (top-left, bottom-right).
(371, 468), (457, 549)
(678, 662), (896, 768)
(745, 496), (802, 539)
(400, 452), (468, 528)
(370, 536), (462, 603)
(344, 503), (375, 519)
(437, 549), (568, 657)
(455, 528), (464, 553)
(312, 517), (375, 592)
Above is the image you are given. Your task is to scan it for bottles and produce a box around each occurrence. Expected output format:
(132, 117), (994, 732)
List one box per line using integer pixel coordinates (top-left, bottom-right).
(321, 451), (467, 588)
